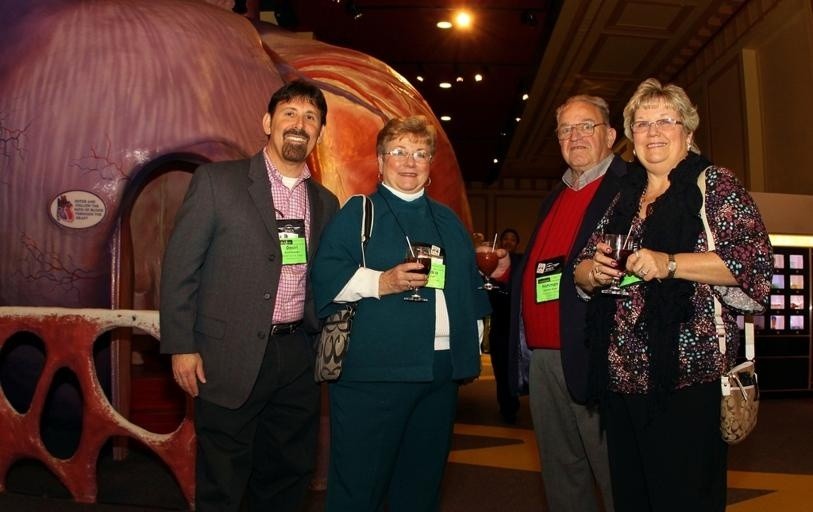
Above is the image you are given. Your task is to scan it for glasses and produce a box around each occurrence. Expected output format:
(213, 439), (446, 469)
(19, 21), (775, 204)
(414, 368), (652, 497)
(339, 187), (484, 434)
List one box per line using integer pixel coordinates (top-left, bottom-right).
(384, 150), (431, 162)
(629, 119), (683, 133)
(557, 122), (607, 140)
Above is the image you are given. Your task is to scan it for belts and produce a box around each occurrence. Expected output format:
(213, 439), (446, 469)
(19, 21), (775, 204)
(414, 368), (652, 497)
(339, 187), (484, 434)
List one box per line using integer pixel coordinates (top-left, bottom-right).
(268, 319), (303, 337)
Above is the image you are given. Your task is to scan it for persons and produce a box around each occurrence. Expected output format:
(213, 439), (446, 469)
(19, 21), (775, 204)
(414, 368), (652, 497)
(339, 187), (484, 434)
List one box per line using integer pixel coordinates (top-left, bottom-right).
(488, 229), (520, 426)
(571, 78), (775, 512)
(159, 79), (341, 512)
(476, 95), (643, 512)
(310, 116), (493, 512)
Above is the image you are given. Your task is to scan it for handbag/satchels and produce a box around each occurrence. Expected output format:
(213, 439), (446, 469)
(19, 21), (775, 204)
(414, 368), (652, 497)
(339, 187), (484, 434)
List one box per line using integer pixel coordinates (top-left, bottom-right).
(722, 361), (760, 448)
(314, 310), (355, 383)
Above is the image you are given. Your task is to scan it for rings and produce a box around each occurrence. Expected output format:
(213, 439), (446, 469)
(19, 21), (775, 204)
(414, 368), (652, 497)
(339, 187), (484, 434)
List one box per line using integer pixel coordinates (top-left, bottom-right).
(635, 251), (638, 258)
(407, 280), (413, 290)
(640, 269), (647, 274)
(594, 265), (601, 276)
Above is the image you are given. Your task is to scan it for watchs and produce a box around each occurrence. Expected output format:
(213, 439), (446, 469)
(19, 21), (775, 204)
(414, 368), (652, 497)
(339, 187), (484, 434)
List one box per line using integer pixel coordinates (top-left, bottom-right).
(668, 254), (677, 279)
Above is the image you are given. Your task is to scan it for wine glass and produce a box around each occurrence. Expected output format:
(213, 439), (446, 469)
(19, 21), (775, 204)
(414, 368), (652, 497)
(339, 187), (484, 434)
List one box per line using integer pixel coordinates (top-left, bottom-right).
(473, 241), (500, 290)
(601, 233), (633, 296)
(403, 246), (430, 302)
